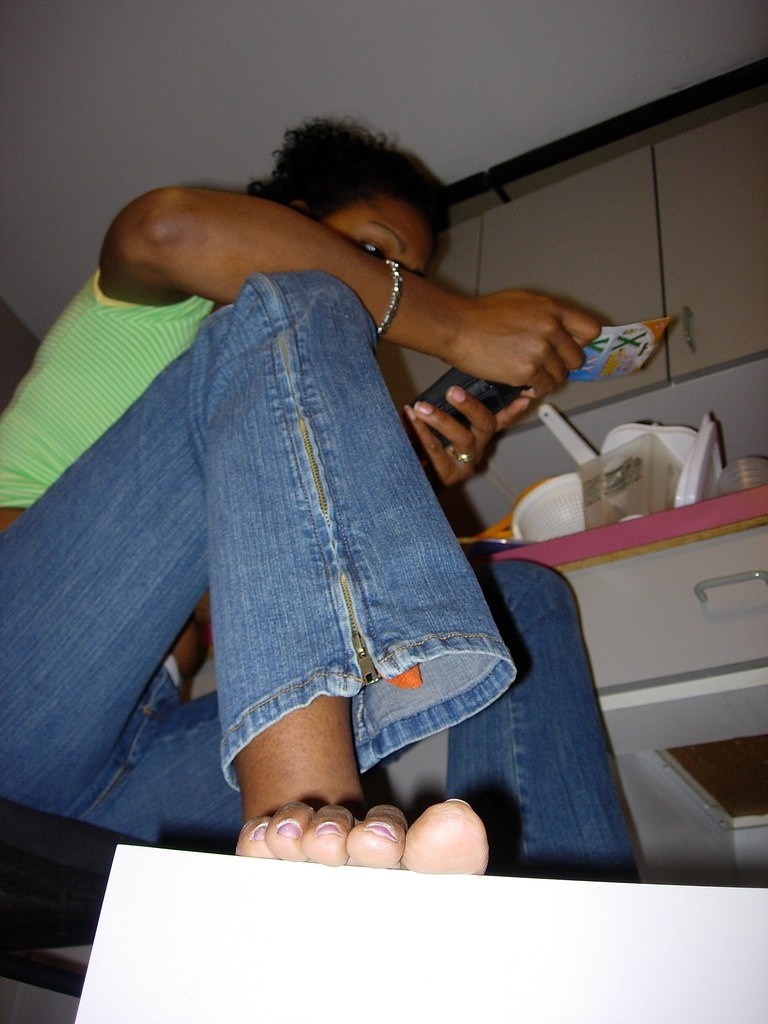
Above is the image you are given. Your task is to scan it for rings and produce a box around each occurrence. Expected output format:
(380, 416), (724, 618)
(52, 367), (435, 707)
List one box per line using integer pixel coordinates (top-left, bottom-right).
(458, 450), (477, 462)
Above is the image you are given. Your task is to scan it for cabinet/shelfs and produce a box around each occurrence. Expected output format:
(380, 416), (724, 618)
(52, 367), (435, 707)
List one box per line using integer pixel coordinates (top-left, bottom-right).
(377, 109), (768, 539)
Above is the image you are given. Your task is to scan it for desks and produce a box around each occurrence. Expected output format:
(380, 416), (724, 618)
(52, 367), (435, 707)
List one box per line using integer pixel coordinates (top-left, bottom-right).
(184, 485), (768, 886)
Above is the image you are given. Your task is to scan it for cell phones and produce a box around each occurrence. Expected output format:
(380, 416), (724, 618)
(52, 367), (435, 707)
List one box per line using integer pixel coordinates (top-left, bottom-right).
(423, 378), (530, 448)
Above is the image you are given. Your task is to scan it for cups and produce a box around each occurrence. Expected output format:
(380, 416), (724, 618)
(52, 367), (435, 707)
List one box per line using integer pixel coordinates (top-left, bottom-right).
(714, 455), (768, 496)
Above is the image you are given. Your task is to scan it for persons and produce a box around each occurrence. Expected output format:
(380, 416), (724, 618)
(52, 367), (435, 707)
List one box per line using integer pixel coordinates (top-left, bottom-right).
(1, 114), (641, 884)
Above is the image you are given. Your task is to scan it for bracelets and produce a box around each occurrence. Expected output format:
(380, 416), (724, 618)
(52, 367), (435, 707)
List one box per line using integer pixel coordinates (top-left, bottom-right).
(376, 259), (404, 334)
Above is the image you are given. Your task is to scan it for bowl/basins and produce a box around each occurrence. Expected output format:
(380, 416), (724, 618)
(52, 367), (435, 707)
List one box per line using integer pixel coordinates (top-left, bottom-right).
(576, 434), (682, 535)
(511, 471), (586, 540)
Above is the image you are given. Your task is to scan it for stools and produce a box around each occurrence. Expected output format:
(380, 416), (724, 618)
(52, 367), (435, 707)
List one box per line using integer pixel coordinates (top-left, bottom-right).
(0, 800), (156, 998)
